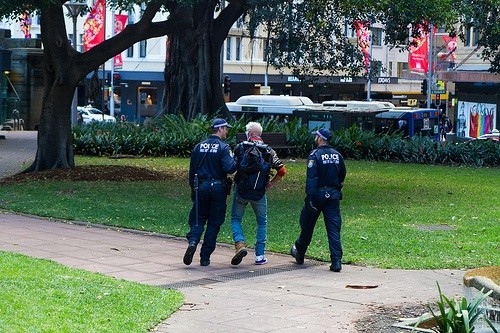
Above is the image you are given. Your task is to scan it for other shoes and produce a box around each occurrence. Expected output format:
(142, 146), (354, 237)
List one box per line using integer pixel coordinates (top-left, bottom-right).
(183, 241), (197, 265)
(291, 245), (304, 264)
(231, 249), (247, 265)
(330, 264), (341, 272)
(255, 255), (268, 264)
(200, 258), (210, 266)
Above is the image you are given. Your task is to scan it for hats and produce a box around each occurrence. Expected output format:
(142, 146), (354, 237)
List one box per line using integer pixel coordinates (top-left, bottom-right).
(210, 118), (232, 129)
(312, 128), (334, 141)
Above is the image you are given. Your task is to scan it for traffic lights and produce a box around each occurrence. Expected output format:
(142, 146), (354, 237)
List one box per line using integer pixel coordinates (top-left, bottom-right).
(224, 75), (231, 95)
(435, 94), (441, 106)
(421, 79), (427, 95)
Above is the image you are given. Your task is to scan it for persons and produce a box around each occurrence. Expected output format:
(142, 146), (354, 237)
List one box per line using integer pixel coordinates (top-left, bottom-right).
(183, 118), (237, 266)
(291, 127), (346, 271)
(230, 121), (286, 265)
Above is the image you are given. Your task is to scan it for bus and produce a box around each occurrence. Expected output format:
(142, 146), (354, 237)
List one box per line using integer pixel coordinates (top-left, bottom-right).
(225, 96), (442, 142)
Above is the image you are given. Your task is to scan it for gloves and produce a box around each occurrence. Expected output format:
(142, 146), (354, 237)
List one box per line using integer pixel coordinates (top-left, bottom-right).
(304, 197), (317, 214)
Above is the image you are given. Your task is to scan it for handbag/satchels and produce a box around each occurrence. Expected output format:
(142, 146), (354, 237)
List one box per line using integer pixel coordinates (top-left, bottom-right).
(234, 141), (273, 201)
(243, 146), (264, 175)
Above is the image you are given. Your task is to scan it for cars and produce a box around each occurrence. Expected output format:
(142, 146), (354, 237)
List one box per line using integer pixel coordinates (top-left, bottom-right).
(77, 106), (116, 124)
